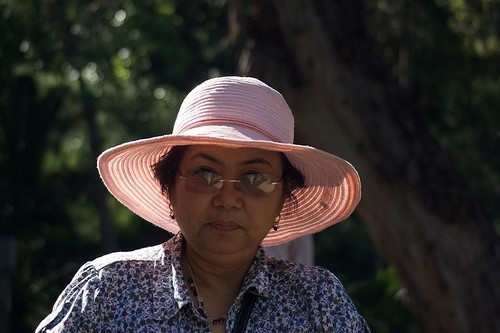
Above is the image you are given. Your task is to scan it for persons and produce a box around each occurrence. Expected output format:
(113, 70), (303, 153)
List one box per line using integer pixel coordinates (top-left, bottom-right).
(34, 76), (371, 333)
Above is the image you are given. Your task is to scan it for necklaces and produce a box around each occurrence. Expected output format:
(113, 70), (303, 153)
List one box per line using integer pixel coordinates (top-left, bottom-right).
(186, 261), (228, 325)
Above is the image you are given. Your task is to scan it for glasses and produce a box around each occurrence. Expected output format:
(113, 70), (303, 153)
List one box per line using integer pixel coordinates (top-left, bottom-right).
(173, 165), (285, 198)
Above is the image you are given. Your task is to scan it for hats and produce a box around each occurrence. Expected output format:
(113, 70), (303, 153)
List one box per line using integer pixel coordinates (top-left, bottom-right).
(97, 76), (362, 248)
